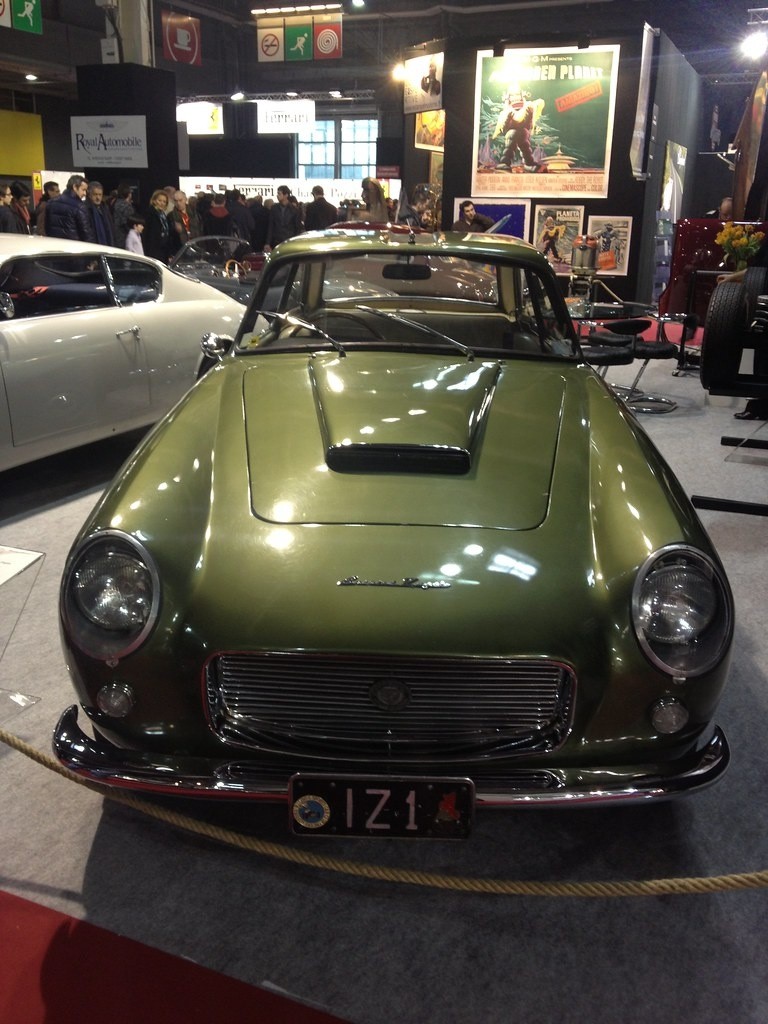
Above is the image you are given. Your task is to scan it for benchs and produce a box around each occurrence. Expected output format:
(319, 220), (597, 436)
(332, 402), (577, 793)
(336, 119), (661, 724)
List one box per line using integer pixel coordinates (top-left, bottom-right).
(310, 310), (516, 348)
(29, 284), (154, 315)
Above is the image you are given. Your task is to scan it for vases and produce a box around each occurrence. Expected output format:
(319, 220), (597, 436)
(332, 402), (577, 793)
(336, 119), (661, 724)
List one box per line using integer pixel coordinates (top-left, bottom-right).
(737, 260), (747, 272)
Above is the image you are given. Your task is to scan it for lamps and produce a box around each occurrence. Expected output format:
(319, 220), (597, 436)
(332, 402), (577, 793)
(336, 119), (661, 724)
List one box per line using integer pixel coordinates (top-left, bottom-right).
(578, 32), (591, 48)
(493, 40), (506, 57)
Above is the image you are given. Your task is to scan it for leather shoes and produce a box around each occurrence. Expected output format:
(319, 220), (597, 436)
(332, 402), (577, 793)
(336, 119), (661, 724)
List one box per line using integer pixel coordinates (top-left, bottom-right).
(734, 400), (768, 421)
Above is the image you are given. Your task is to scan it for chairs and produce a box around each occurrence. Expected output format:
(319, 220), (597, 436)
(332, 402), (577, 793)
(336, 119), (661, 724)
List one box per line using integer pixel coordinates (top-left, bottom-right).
(578, 301), (689, 413)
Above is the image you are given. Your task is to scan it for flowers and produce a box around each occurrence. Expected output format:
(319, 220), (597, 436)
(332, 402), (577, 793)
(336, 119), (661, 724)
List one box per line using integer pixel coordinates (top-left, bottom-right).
(715, 221), (765, 265)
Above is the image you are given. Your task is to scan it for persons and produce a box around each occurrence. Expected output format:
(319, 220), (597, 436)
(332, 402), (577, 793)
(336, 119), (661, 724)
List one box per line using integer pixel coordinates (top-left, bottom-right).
(421, 65), (441, 96)
(450, 201), (494, 233)
(543, 216), (566, 263)
(0, 175), (398, 265)
(717, 231), (768, 420)
(703, 197), (733, 220)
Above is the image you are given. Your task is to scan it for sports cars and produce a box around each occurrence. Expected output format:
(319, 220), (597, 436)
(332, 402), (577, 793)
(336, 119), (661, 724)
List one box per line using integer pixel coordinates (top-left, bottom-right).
(50, 230), (733, 842)
(0, 230), (317, 476)
(167, 236), (498, 315)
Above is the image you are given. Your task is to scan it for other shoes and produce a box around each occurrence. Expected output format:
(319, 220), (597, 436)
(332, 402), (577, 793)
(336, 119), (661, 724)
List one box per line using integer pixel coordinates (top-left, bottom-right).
(679, 326), (697, 340)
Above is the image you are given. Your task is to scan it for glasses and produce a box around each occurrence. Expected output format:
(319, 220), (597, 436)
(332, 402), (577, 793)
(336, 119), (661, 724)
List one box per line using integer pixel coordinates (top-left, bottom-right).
(4, 192), (12, 196)
